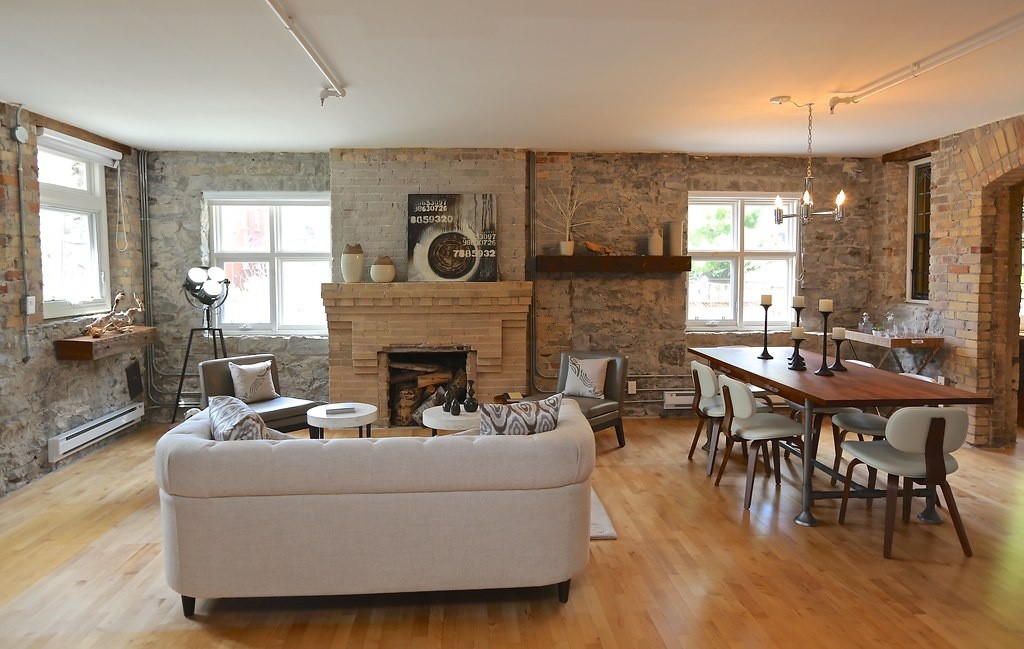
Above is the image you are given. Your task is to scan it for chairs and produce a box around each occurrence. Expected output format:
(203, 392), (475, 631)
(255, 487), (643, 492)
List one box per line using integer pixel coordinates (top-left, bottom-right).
(197, 354), (321, 439)
(687, 358), (973, 558)
(519, 351), (630, 446)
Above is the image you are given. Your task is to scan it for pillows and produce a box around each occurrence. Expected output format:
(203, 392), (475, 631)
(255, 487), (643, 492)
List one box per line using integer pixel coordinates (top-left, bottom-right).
(228, 359), (280, 403)
(481, 393), (563, 436)
(562, 355), (612, 399)
(208, 396), (272, 441)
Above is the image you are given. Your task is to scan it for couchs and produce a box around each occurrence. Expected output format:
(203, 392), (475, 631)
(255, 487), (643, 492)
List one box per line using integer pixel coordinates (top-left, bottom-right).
(155, 399), (596, 617)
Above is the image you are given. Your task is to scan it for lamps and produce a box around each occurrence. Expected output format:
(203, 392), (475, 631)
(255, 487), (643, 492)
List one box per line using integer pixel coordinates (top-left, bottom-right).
(183, 263), (230, 310)
(770, 97), (845, 223)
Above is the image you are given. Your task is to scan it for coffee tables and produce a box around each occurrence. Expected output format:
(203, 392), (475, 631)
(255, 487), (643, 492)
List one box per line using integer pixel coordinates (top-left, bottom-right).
(306, 403), (379, 439)
(423, 404), (481, 435)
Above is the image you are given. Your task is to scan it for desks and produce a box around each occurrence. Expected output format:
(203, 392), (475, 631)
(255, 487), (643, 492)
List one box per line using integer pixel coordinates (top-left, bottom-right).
(846, 327), (943, 375)
(688, 347), (994, 526)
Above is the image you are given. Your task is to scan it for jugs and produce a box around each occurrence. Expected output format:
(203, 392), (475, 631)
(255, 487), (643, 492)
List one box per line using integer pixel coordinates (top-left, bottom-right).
(648, 227), (664, 256)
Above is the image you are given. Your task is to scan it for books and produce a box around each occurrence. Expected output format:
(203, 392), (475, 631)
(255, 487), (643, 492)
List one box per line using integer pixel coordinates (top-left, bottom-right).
(326, 407), (355, 414)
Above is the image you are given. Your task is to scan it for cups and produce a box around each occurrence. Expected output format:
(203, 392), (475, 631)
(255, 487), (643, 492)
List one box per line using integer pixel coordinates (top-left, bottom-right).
(668, 220), (684, 257)
(341, 253), (363, 282)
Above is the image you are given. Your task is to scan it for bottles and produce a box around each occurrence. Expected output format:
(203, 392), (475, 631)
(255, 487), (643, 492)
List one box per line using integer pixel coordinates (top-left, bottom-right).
(858, 312), (873, 334)
(883, 311), (896, 332)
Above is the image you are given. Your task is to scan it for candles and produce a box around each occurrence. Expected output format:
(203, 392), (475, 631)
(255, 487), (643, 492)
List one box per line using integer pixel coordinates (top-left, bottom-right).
(793, 296), (804, 307)
(819, 299), (834, 311)
(791, 327), (805, 339)
(761, 295), (772, 304)
(832, 327), (845, 339)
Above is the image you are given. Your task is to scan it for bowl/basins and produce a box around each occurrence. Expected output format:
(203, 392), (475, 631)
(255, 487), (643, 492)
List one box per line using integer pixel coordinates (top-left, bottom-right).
(370, 264), (395, 283)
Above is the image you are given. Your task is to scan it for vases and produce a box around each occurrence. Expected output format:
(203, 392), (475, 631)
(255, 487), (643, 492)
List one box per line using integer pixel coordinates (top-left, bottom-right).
(560, 241), (574, 256)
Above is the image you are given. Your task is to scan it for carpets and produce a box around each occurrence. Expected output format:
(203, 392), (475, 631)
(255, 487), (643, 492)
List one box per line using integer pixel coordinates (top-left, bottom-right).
(591, 484), (617, 540)
